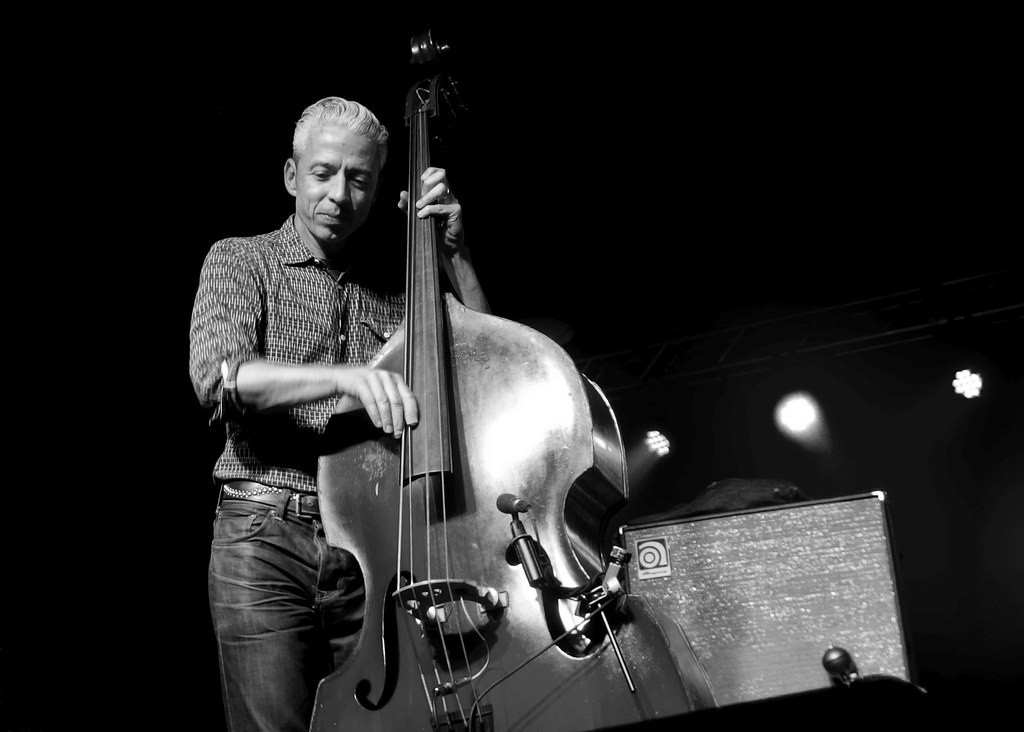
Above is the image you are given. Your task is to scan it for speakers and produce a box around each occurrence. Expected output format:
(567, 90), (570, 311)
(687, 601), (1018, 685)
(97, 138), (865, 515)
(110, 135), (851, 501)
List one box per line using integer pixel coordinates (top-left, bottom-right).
(618, 491), (914, 711)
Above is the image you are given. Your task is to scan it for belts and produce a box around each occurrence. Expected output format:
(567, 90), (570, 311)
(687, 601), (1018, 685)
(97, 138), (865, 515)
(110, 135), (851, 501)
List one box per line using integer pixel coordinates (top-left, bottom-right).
(221, 480), (321, 519)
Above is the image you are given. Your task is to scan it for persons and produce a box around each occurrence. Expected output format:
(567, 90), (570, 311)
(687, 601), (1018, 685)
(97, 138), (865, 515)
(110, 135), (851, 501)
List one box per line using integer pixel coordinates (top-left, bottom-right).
(188, 96), (484, 732)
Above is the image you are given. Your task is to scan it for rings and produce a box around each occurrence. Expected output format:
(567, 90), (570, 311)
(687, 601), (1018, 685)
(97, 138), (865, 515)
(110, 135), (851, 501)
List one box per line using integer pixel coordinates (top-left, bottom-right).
(441, 189), (450, 199)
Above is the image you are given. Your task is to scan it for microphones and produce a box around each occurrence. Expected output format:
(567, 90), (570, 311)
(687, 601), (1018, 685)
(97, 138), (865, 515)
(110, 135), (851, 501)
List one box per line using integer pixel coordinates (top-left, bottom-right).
(822, 647), (860, 686)
(497, 493), (532, 514)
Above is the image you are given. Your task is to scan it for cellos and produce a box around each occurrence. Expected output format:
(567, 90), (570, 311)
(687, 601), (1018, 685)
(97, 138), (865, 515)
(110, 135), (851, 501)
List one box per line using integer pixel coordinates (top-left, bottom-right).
(306, 28), (720, 731)
